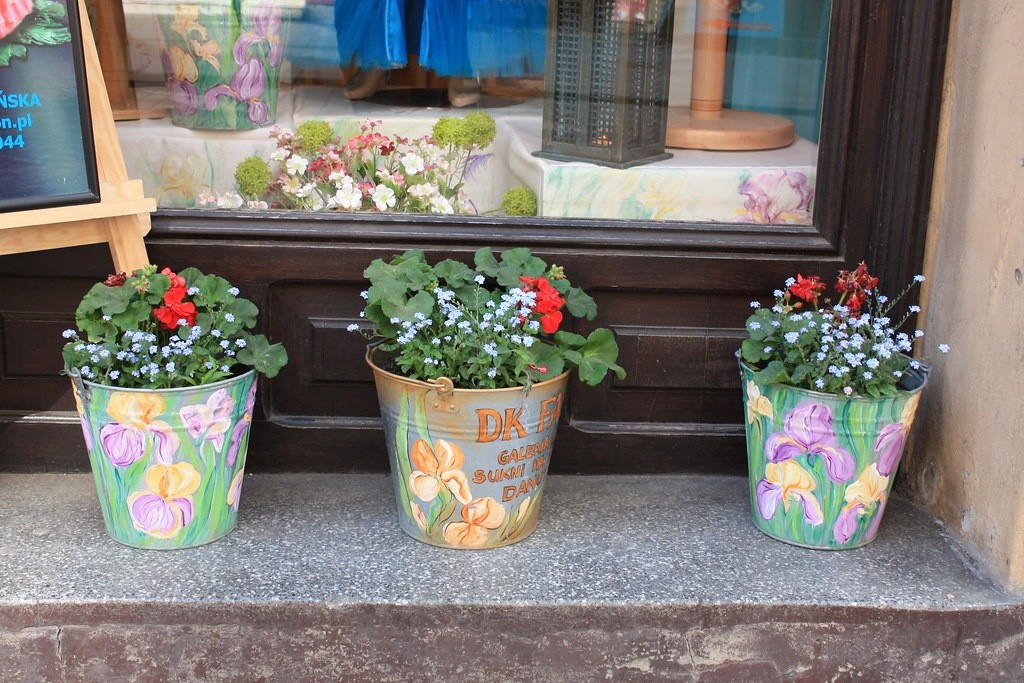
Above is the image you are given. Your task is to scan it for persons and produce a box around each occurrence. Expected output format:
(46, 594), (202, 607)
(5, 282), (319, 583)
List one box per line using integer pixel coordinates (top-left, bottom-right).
(332, 0), (549, 107)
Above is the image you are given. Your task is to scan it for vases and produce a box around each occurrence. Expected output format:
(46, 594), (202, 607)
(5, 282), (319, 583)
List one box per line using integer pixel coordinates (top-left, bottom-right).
(62, 363), (257, 549)
(365, 337), (571, 548)
(735, 350), (932, 551)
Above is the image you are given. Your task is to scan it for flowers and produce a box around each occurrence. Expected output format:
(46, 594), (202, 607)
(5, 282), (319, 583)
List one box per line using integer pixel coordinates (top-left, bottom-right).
(743, 260), (949, 397)
(61, 264), (289, 391)
(347, 249), (626, 395)
(198, 112), (539, 216)
